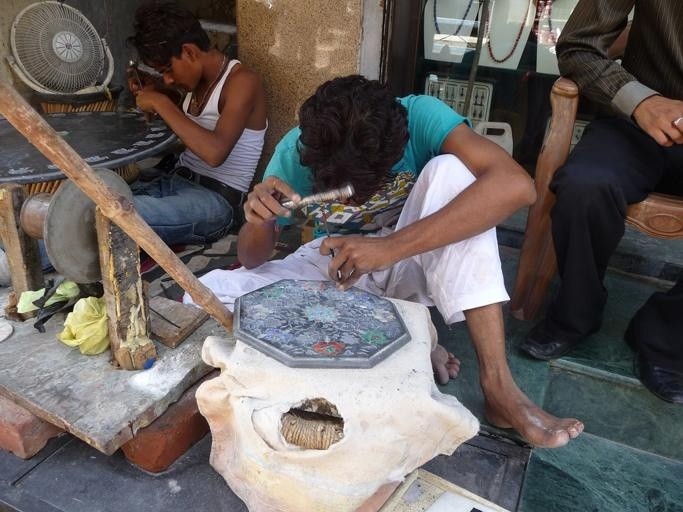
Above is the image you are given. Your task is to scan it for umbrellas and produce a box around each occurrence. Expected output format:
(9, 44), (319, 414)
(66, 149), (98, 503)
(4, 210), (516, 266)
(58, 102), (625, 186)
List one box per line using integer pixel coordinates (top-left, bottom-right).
(520, 317), (602, 359)
(624, 330), (683, 404)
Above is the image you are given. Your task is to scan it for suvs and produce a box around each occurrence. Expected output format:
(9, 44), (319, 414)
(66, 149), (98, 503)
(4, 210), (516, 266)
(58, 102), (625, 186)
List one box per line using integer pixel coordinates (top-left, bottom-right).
(473, 122), (514, 156)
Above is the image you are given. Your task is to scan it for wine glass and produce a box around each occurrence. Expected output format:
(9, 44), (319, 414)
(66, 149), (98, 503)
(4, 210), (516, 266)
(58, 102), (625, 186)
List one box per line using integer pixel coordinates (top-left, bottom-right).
(672, 117), (683, 127)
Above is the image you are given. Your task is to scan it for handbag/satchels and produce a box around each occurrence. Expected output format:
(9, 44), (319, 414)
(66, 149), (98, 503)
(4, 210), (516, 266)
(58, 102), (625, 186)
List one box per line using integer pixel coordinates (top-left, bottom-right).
(174, 86), (186, 107)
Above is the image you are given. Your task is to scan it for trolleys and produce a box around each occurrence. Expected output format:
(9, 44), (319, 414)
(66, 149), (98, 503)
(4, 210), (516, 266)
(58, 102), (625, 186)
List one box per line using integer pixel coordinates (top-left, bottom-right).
(5, 1), (115, 96)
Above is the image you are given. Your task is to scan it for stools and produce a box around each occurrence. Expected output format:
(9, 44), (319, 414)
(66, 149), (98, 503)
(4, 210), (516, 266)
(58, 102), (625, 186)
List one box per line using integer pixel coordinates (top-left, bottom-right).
(177, 166), (246, 206)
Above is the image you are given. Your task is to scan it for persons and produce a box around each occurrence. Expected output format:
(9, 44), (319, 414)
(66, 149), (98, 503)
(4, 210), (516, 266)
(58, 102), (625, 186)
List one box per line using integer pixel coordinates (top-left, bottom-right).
(237, 74), (585, 449)
(520, 0), (683, 404)
(0, 0), (270, 288)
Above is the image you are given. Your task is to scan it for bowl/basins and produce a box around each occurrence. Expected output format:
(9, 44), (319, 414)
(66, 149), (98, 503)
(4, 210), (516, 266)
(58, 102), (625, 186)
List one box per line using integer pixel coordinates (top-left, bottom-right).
(546, 0), (558, 46)
(484, 0), (529, 62)
(432, 0), (472, 39)
(188, 53), (227, 116)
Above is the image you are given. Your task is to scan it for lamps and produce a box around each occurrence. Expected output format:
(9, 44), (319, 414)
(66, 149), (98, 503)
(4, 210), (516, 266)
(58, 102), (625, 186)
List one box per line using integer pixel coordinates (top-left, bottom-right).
(508, 19), (683, 322)
